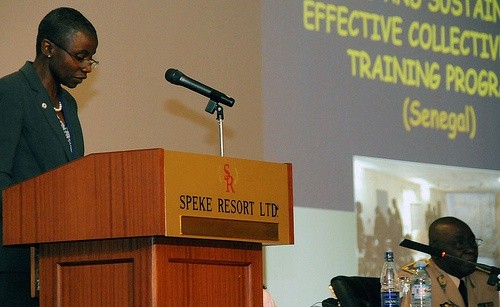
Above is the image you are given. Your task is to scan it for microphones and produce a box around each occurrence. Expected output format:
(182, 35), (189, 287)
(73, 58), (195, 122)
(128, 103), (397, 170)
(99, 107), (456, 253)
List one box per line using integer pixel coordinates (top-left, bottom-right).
(399, 239), (500, 274)
(164, 68), (235, 107)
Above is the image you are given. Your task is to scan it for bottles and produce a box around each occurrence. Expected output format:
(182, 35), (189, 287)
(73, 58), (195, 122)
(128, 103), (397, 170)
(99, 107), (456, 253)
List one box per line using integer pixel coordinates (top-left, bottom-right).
(410, 261), (432, 307)
(380, 252), (401, 307)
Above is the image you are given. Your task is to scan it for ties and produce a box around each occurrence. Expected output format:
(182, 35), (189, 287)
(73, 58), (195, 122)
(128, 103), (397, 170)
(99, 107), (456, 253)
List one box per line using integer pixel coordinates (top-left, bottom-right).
(458, 280), (468, 307)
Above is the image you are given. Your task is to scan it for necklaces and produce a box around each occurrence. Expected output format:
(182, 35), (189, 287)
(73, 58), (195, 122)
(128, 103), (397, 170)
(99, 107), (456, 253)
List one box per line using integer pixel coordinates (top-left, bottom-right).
(53, 101), (62, 111)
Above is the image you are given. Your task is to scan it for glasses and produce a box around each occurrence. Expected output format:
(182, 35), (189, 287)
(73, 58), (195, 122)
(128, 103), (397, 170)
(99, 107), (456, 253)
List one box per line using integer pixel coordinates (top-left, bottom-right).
(48, 40), (99, 69)
(436, 238), (483, 249)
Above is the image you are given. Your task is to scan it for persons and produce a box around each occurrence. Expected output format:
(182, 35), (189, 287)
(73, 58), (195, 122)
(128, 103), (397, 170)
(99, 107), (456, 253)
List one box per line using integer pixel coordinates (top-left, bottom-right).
(0, 8), (99, 307)
(424, 200), (441, 231)
(356, 196), (403, 256)
(399, 216), (500, 307)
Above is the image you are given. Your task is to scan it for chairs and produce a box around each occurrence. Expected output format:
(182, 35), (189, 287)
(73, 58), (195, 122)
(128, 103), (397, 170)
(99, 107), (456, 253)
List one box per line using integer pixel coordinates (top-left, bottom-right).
(329, 273), (384, 307)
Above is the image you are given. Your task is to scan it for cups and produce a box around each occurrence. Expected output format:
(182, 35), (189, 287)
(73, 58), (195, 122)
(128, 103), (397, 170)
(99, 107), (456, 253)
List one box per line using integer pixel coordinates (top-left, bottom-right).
(398, 275), (411, 307)
